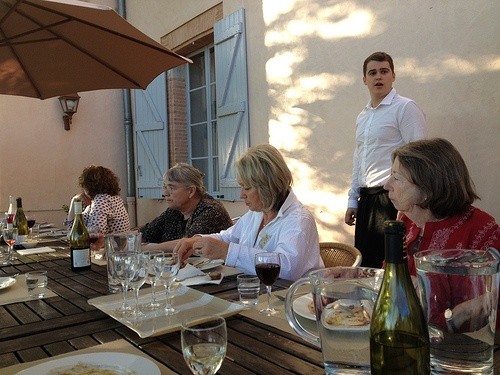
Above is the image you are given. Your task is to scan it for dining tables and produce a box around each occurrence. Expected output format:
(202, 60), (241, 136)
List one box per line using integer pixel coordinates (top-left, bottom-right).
(0, 217), (495, 375)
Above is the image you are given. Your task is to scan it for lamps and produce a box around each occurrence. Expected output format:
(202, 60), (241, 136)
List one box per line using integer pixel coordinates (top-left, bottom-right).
(58, 92), (81, 129)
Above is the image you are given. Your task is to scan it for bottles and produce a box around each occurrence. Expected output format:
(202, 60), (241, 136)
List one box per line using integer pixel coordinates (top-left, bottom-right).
(6, 195), (16, 229)
(369, 219), (431, 375)
(13, 197), (27, 250)
(69, 199), (91, 273)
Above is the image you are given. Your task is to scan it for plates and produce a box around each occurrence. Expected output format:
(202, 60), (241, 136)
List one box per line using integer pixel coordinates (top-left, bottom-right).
(184, 257), (225, 270)
(0, 277), (17, 290)
(88, 283), (243, 339)
(13, 351), (161, 375)
(292, 292), (317, 321)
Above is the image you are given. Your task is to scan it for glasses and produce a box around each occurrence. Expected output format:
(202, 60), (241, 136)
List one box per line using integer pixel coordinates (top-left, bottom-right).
(161, 185), (191, 194)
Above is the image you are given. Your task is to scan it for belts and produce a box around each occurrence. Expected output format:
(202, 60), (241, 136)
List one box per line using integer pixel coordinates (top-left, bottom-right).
(359, 188), (384, 195)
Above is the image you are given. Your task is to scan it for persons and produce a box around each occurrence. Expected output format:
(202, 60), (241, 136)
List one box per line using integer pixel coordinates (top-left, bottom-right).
(127, 163), (234, 257)
(64, 164), (131, 250)
(377, 137), (500, 333)
(344, 51), (429, 268)
(173, 144), (325, 281)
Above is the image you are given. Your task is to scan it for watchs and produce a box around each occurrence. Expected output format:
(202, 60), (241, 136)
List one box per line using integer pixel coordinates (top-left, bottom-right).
(444, 309), (457, 331)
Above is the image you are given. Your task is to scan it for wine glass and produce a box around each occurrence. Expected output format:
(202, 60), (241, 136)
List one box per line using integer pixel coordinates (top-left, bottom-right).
(2, 228), (18, 260)
(254, 252), (282, 317)
(25, 214), (36, 239)
(111, 248), (180, 324)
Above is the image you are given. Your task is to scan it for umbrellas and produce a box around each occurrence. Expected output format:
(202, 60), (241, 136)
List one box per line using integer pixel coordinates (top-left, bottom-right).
(0, 0), (194, 100)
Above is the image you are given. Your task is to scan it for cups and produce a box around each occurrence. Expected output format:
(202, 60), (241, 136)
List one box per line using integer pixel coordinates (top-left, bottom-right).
(237, 273), (260, 305)
(87, 226), (103, 251)
(180, 313), (228, 375)
(413, 249), (500, 375)
(284, 266), (385, 375)
(25, 270), (48, 299)
(105, 231), (141, 294)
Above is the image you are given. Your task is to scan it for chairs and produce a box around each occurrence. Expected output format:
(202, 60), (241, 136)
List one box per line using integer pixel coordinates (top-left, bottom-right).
(317, 241), (362, 272)
